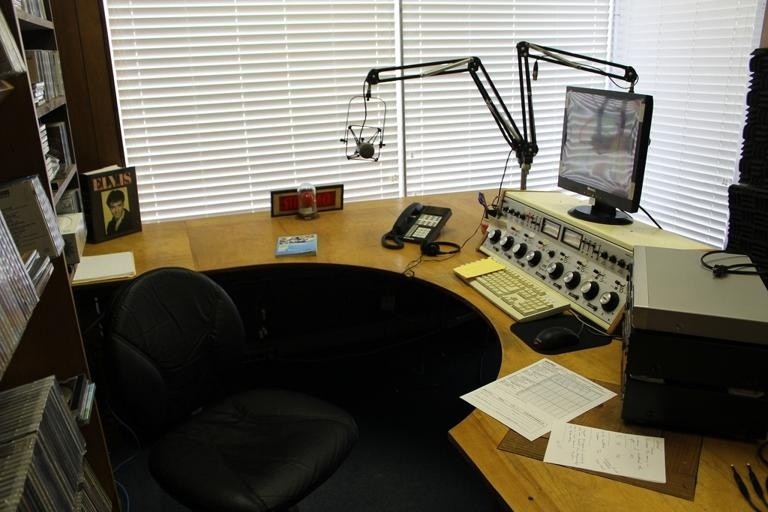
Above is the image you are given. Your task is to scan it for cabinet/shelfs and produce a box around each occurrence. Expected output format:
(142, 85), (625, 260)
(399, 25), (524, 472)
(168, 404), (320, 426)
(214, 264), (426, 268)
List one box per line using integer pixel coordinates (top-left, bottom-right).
(0, 0), (122, 512)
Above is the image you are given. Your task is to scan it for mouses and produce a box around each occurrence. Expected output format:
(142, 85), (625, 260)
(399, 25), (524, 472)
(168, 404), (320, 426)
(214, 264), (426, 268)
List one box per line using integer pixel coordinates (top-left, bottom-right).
(532, 326), (581, 347)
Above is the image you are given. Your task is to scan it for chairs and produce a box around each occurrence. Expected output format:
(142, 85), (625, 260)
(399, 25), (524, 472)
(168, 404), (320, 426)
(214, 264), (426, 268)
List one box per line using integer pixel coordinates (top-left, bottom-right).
(108, 266), (359, 511)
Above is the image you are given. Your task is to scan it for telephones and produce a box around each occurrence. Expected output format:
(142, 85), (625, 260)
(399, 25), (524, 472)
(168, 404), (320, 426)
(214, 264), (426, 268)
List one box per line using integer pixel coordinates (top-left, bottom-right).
(390, 201), (453, 245)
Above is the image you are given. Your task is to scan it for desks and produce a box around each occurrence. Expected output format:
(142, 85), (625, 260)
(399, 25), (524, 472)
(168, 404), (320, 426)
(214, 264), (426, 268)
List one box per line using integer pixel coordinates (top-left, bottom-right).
(70, 187), (768, 511)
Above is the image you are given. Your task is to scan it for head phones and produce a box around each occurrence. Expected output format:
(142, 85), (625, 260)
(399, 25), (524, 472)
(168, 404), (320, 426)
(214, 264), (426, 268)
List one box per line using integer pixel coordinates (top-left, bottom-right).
(420, 238), (461, 256)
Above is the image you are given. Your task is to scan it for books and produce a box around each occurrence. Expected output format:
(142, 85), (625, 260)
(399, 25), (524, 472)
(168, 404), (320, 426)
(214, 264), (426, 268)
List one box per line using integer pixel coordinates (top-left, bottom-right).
(274, 233), (318, 257)
(0, 0), (114, 512)
(79, 163), (142, 244)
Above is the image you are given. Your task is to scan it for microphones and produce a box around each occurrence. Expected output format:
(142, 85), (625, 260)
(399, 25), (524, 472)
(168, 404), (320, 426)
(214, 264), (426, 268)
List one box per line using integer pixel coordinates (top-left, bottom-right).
(356, 142), (375, 159)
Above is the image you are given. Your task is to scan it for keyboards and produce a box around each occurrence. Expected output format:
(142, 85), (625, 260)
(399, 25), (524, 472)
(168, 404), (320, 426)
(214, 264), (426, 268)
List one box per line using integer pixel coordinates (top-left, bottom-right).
(453, 256), (572, 322)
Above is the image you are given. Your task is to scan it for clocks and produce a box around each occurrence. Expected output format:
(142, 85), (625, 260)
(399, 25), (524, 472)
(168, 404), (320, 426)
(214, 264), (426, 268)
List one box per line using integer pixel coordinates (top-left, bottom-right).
(269, 182), (345, 218)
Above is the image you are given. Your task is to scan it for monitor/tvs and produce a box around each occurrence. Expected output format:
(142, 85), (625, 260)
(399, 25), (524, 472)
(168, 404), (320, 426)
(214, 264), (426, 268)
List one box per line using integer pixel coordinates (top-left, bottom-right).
(556, 85), (654, 225)
